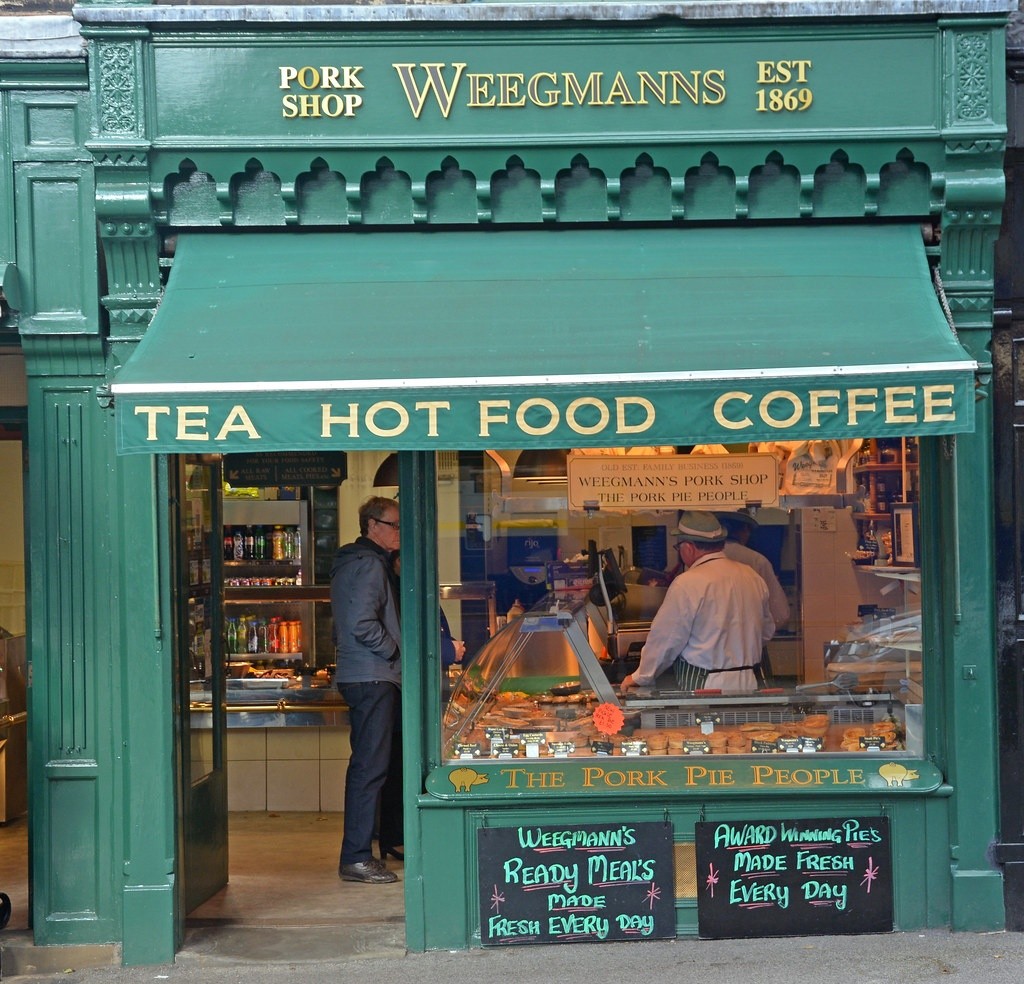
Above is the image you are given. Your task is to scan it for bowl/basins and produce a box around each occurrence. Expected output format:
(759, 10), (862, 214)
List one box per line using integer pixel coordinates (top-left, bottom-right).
(227, 662), (252, 677)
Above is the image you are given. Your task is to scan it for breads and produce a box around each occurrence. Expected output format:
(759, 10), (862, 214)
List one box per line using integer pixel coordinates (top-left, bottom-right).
(840, 722), (898, 751)
(455, 680), (829, 758)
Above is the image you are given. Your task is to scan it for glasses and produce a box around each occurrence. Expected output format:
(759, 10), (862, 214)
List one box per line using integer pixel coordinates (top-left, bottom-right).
(673, 540), (692, 551)
(373, 518), (399, 530)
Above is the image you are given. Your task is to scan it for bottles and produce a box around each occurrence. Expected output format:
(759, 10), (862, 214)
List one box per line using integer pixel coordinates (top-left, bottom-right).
(224, 614), (303, 654)
(877, 477), (888, 512)
(187, 598), (212, 679)
(223, 525), (301, 560)
(189, 526), (212, 587)
(860, 440), (871, 464)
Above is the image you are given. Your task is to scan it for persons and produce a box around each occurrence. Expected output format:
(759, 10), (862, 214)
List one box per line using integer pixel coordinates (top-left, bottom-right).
(329, 496), (403, 884)
(377, 541), (468, 862)
(620, 509), (774, 694)
(712, 510), (792, 626)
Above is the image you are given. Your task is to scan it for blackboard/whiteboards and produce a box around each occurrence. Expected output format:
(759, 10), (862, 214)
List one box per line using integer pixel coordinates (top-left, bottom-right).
(694, 815), (895, 940)
(477, 822), (678, 946)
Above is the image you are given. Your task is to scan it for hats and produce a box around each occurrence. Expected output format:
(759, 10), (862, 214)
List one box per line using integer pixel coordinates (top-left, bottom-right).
(670, 511), (728, 542)
(713, 508), (759, 528)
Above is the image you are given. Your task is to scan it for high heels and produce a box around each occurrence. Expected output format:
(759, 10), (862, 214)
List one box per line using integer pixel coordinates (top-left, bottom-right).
(380, 848), (404, 860)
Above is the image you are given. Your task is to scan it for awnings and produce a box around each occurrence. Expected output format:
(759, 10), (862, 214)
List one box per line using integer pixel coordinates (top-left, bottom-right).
(108, 229), (978, 452)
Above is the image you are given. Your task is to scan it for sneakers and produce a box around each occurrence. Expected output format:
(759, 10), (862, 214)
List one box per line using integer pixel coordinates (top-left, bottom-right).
(339, 856), (398, 883)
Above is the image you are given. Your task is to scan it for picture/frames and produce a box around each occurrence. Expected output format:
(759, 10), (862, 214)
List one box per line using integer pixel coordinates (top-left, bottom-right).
(889, 502), (920, 568)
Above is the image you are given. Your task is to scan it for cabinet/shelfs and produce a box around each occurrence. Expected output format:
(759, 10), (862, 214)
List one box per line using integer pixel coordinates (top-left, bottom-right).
(852, 437), (920, 567)
(0, 634), (27, 825)
